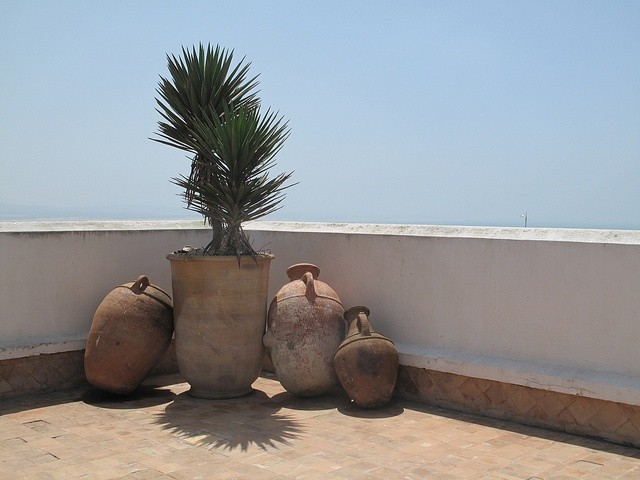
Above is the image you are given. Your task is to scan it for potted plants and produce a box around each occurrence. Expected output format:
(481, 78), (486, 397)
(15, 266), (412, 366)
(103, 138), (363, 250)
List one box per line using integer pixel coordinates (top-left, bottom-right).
(149, 41), (299, 400)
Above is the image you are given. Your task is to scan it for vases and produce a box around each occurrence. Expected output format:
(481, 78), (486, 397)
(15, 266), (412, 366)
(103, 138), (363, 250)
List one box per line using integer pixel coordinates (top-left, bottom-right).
(84, 274), (173, 395)
(262, 263), (346, 400)
(335, 306), (400, 408)
